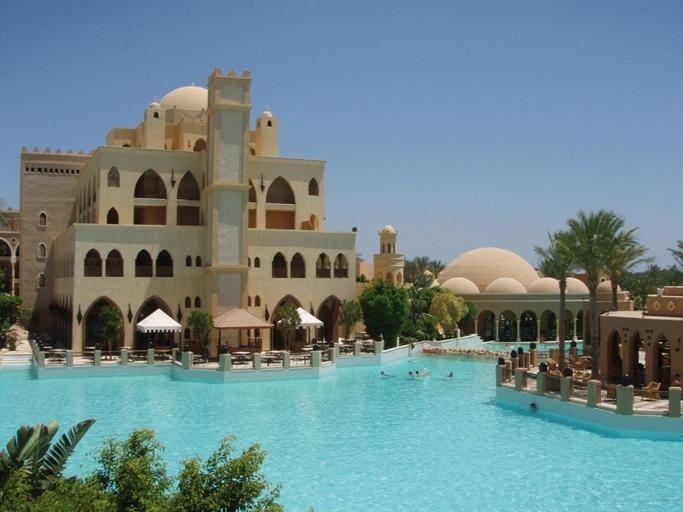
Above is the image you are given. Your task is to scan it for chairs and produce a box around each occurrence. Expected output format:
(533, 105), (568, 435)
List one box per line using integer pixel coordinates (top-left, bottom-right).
(545, 352), (661, 404)
(32, 332), (375, 367)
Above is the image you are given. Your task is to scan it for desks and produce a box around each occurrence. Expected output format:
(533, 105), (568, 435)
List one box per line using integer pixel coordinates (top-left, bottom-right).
(32, 332), (375, 367)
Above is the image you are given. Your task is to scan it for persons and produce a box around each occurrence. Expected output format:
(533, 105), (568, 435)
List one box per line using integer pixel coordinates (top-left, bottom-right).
(530, 403), (535, 409)
(408, 369), (428, 376)
(449, 372), (453, 377)
(672, 374), (681, 386)
(635, 363), (640, 387)
(570, 348), (577, 367)
(380, 370), (385, 376)
